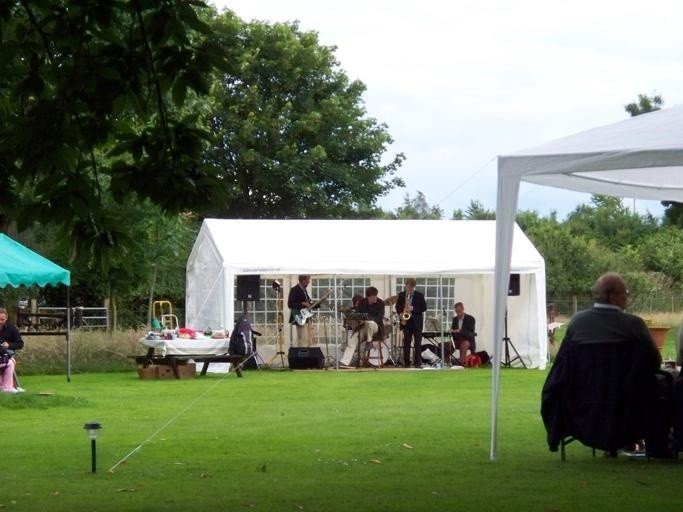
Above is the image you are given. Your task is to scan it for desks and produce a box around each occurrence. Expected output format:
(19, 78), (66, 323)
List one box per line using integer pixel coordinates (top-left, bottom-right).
(138, 337), (230, 377)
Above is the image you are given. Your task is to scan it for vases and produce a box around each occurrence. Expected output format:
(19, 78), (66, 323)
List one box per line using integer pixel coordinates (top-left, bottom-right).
(645, 326), (671, 352)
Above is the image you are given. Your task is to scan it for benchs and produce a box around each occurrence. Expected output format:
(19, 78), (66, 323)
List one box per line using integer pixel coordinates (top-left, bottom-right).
(135, 354), (245, 379)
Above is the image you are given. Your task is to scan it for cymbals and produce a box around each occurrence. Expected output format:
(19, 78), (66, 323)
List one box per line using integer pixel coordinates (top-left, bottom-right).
(383, 296), (399, 306)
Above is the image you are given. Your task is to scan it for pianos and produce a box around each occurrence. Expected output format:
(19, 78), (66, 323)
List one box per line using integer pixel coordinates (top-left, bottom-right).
(422, 331), (477, 336)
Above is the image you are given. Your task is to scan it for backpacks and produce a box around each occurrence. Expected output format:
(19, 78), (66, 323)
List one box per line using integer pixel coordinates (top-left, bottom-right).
(229, 316), (254, 354)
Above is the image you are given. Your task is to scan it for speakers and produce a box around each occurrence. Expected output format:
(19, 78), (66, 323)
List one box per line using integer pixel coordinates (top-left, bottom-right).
(508, 274), (520, 296)
(236, 275), (260, 302)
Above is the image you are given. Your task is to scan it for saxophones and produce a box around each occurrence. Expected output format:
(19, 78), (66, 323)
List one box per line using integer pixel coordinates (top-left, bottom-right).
(401, 293), (410, 325)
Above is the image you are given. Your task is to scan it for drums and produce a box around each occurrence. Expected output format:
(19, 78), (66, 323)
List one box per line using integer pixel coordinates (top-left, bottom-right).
(365, 341), (389, 367)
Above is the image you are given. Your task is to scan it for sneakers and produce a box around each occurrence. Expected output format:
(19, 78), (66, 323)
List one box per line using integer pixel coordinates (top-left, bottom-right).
(366, 342), (373, 351)
(338, 362), (346, 366)
(406, 362), (423, 368)
(7, 387), (24, 394)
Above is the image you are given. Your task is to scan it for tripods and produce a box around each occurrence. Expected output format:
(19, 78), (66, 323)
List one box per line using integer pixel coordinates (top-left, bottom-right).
(226, 301), (266, 372)
(481, 306), (527, 370)
(269, 293), (289, 368)
(319, 317), (346, 371)
(387, 306), (398, 366)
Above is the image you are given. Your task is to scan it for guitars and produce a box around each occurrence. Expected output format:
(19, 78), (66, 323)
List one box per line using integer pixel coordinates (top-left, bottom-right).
(294, 286), (333, 326)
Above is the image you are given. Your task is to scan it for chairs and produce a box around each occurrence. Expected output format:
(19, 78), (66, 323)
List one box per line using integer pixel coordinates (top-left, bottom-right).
(560, 339), (673, 461)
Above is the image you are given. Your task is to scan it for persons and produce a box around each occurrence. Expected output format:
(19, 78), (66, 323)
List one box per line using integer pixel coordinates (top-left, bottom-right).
(332, 286), (384, 368)
(346, 294), (363, 343)
(562, 271), (680, 462)
(287, 274), (326, 348)
(442, 301), (475, 365)
(394, 278), (427, 368)
(0, 307), (23, 389)
